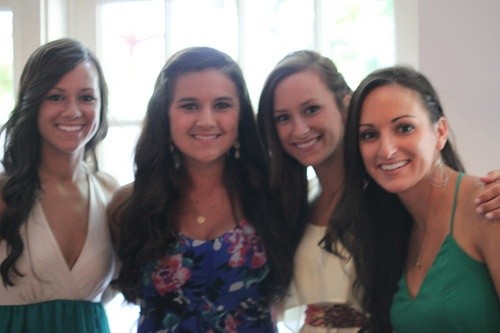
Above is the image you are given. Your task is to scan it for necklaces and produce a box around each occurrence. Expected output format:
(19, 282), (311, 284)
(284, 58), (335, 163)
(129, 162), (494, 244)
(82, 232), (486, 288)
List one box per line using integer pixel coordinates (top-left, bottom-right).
(402, 162), (449, 270)
(180, 184), (229, 225)
(317, 173), (344, 226)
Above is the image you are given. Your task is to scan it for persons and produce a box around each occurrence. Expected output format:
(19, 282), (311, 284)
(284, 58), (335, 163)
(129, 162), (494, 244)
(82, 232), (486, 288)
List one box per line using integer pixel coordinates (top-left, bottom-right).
(0, 38), (125, 333)
(105, 47), (295, 333)
(255, 50), (500, 333)
(317, 64), (500, 333)
